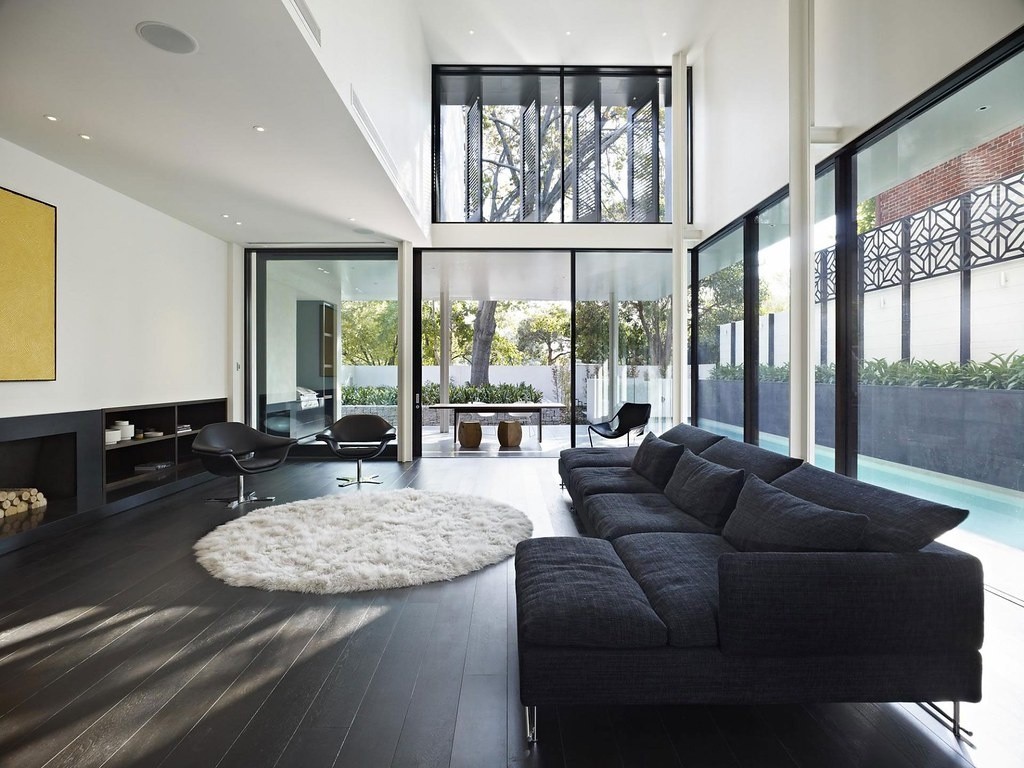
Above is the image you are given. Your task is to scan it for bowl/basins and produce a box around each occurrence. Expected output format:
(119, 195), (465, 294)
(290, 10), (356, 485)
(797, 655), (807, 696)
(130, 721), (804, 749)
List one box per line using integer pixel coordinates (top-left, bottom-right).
(113, 425), (134, 441)
(104, 429), (121, 445)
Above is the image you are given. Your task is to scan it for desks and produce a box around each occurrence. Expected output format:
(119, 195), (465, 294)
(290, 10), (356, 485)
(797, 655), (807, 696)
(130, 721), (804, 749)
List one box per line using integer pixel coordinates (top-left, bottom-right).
(429, 402), (566, 443)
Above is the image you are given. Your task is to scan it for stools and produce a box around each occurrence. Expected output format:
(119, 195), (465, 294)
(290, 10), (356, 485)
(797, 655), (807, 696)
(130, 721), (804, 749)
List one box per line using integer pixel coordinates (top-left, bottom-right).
(498, 420), (522, 446)
(458, 421), (482, 447)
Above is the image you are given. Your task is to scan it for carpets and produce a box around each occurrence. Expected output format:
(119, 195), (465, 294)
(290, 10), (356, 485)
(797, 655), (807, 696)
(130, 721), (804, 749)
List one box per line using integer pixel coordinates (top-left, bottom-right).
(190, 487), (532, 595)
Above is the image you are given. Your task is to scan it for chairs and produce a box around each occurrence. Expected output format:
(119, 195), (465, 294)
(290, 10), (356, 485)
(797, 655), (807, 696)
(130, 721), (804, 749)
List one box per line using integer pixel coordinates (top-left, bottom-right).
(468, 401), (497, 435)
(315, 414), (397, 487)
(191, 422), (298, 509)
(508, 401), (536, 437)
(587, 402), (651, 447)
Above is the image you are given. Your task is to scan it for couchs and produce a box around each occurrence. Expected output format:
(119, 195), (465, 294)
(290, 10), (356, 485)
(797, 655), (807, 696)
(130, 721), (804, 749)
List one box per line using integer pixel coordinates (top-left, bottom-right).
(515, 446), (984, 744)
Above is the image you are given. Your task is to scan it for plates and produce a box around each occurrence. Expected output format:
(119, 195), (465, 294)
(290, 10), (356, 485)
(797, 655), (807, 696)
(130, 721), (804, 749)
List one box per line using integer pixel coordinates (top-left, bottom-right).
(144, 431), (164, 437)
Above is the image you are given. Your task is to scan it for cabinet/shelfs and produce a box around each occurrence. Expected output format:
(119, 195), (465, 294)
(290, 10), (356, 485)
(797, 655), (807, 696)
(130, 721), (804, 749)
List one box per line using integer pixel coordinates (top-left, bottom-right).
(266, 398), (325, 441)
(297, 299), (337, 429)
(101, 397), (228, 518)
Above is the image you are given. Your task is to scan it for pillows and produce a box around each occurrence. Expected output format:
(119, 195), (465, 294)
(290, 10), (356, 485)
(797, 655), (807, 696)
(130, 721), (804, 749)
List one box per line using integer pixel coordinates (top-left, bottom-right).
(657, 422), (727, 455)
(699, 438), (802, 484)
(631, 430), (684, 490)
(664, 447), (746, 528)
(768, 461), (970, 552)
(722, 472), (869, 551)
